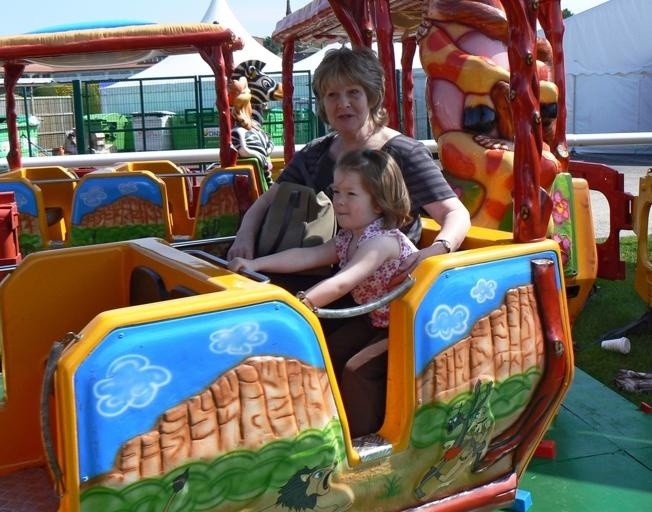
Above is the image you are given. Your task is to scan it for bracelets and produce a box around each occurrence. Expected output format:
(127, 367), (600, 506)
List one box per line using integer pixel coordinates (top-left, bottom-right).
(296, 288), (320, 316)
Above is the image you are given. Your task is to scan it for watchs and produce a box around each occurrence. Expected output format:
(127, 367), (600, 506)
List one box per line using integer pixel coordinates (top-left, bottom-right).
(431, 237), (453, 254)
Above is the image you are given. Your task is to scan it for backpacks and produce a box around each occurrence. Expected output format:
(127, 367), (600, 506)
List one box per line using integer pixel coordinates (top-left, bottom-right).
(246, 152), (339, 278)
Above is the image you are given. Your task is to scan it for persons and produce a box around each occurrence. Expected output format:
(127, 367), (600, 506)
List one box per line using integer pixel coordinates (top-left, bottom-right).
(225, 47), (472, 440)
(225, 147), (422, 442)
(230, 93), (274, 189)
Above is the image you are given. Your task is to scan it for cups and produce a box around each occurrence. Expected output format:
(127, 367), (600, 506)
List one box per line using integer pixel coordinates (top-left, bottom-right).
(600, 337), (631, 354)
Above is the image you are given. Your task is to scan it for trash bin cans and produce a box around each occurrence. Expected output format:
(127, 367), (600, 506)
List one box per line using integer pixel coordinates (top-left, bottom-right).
(81, 108), (318, 153)
(0, 115), (40, 159)
